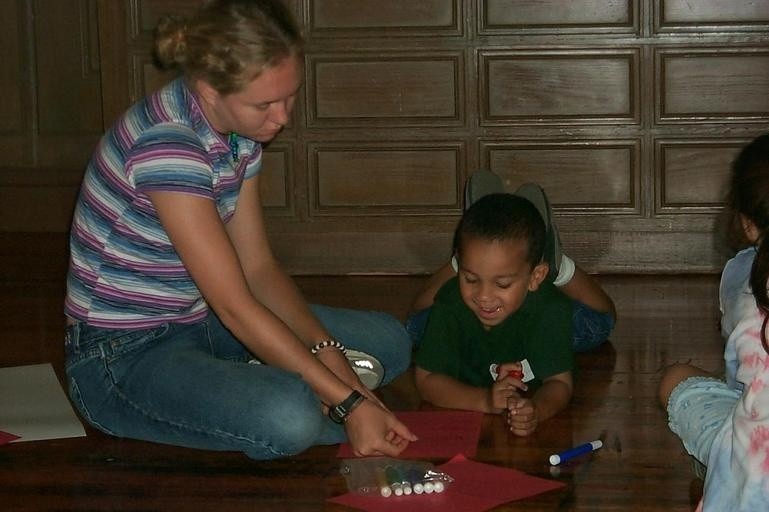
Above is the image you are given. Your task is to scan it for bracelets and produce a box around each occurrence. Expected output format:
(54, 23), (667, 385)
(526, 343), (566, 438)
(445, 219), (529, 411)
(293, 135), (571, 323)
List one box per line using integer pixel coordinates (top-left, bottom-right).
(309, 340), (347, 358)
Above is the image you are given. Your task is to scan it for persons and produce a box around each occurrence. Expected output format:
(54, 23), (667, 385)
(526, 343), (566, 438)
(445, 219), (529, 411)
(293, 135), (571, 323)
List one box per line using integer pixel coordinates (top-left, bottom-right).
(408, 167), (617, 439)
(660, 134), (768, 512)
(50, 1), (415, 465)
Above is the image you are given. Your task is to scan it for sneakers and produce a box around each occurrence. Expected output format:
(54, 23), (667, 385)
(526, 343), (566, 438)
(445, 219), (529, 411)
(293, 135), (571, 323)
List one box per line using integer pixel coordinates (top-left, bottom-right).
(240, 340), (381, 397)
(454, 171), (504, 215)
(512, 170), (566, 275)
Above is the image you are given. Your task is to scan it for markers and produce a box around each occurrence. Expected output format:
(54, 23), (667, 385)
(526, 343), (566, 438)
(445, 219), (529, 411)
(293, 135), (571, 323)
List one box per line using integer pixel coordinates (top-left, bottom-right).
(549, 439), (603, 467)
(490, 364), (525, 381)
(374, 461), (445, 498)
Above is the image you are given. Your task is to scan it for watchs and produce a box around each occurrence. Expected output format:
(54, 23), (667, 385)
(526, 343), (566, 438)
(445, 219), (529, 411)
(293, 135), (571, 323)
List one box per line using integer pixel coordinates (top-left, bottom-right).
(326, 386), (368, 426)
